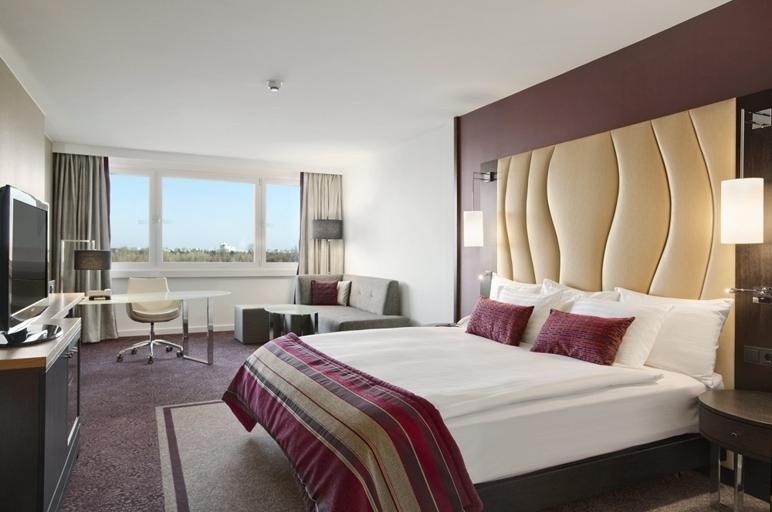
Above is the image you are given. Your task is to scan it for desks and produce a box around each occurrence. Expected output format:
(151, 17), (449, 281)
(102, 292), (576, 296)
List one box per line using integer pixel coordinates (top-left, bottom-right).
(76, 287), (231, 365)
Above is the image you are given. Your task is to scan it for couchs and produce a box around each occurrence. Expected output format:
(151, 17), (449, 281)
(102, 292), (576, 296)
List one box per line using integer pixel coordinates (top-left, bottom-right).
(233, 272), (413, 345)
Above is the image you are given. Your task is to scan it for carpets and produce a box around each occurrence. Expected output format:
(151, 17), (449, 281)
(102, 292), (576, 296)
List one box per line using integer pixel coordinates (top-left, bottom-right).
(155, 398), (306, 511)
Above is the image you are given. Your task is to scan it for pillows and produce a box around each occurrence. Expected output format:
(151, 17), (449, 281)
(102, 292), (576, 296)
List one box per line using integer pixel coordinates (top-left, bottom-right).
(308, 276), (348, 305)
(464, 274), (734, 374)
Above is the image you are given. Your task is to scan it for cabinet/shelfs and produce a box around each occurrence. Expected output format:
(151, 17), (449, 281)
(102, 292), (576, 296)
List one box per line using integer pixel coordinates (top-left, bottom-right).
(1, 291), (85, 511)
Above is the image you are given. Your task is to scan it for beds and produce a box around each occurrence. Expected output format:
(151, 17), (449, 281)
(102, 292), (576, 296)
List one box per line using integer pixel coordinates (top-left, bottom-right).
(252, 95), (737, 511)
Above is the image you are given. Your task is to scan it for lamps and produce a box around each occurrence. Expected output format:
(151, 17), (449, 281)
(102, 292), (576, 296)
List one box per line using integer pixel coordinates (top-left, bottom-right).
(462, 157), (497, 246)
(718, 102), (771, 246)
(311, 215), (344, 271)
(58, 238), (112, 294)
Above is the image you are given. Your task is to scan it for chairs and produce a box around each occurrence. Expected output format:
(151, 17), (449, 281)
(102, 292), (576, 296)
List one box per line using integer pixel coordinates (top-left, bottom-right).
(118, 276), (184, 366)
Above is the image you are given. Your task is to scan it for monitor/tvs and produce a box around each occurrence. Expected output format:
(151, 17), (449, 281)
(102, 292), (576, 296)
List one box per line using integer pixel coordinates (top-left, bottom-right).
(0, 185), (64, 347)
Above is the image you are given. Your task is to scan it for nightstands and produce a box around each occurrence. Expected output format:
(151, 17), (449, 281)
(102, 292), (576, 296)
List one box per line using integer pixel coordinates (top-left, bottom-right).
(697, 387), (771, 511)
